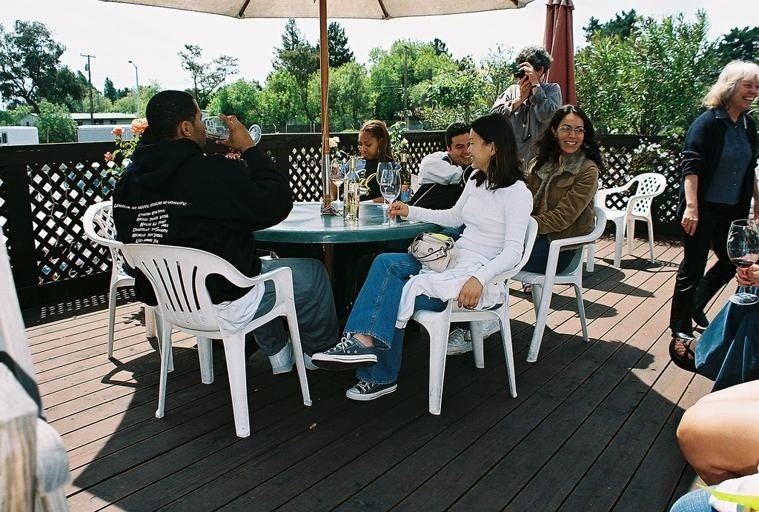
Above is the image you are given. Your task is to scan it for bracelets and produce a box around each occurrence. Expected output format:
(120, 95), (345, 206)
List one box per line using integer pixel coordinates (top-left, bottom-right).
(531, 84), (540, 89)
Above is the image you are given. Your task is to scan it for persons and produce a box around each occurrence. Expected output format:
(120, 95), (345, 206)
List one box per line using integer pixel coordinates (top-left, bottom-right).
(311, 112), (534, 400)
(446, 104), (606, 355)
(403, 121), (470, 210)
(669, 263), (759, 512)
(671, 59), (759, 338)
(329, 121), (403, 203)
(113, 90), (339, 375)
(490, 46), (562, 179)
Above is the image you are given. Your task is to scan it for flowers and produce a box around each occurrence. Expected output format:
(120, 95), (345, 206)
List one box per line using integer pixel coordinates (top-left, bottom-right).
(104, 118), (148, 184)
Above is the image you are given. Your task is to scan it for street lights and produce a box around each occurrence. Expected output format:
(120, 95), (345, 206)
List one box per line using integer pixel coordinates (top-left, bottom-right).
(128, 59), (142, 113)
(401, 43), (412, 124)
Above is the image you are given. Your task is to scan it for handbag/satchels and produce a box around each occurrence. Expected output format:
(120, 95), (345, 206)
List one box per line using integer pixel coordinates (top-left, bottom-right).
(408, 229), (458, 273)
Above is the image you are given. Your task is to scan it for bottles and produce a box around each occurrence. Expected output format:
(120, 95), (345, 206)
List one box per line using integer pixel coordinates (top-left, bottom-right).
(343, 156), (361, 225)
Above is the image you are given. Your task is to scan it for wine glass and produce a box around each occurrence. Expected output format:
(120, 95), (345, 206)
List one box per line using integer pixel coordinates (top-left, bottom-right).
(726, 218), (759, 305)
(327, 157), (346, 210)
(201, 116), (262, 145)
(375, 160), (401, 228)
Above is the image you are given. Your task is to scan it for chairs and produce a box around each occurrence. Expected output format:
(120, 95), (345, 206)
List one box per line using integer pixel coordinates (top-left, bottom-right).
(586, 171), (667, 274)
(409, 217), (538, 416)
(83, 200), (175, 373)
(118, 244), (314, 437)
(509, 206), (608, 363)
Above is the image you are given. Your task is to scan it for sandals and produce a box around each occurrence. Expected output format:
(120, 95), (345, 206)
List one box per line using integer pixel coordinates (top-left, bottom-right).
(668, 338), (699, 374)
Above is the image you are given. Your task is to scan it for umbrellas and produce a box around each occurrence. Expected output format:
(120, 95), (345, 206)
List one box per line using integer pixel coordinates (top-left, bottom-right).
(544, 0), (576, 108)
(101, 0), (535, 286)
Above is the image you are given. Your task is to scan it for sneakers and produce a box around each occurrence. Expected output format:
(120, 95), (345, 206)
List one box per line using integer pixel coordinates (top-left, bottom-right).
(464, 304), (509, 340)
(303, 351), (320, 372)
(446, 328), (480, 357)
(344, 376), (401, 402)
(309, 334), (379, 371)
(672, 323), (696, 339)
(692, 310), (709, 329)
(266, 339), (296, 378)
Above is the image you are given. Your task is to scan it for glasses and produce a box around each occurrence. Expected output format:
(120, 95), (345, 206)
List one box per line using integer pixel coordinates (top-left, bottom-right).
(561, 125), (585, 135)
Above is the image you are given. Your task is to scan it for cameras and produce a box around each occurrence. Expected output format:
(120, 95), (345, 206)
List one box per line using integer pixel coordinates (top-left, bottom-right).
(514, 66), (525, 79)
